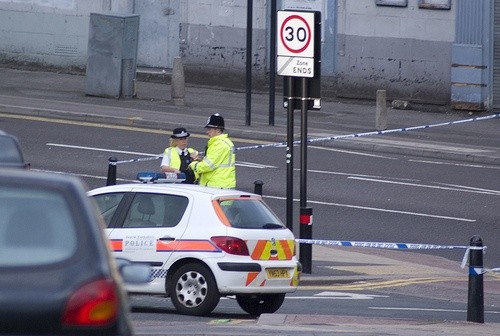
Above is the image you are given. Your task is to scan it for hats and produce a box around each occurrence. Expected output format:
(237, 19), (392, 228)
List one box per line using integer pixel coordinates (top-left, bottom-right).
(170, 127), (190, 138)
(204, 114), (225, 131)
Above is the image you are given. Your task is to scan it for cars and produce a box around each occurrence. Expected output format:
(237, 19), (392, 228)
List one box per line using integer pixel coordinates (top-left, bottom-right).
(1, 166), (134, 336)
(87, 170), (303, 316)
(0, 128), (33, 170)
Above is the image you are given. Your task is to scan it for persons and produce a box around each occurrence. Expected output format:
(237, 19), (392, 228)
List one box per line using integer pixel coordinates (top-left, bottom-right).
(184, 113), (238, 211)
(160, 128), (200, 185)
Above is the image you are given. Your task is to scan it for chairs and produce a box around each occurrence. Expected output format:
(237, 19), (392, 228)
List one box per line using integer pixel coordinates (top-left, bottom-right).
(123, 196), (156, 228)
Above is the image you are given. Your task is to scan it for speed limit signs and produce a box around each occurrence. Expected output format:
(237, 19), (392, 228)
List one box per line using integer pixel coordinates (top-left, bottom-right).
(276, 9), (315, 78)
